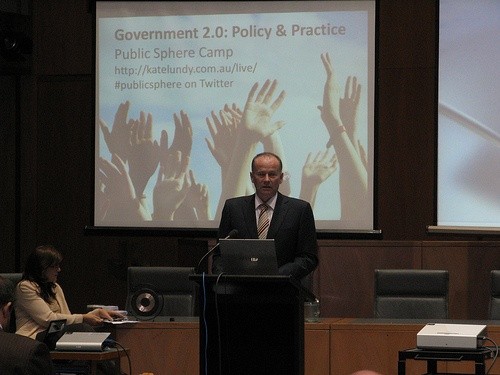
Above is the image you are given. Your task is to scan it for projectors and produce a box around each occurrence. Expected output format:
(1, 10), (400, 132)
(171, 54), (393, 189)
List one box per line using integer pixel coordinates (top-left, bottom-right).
(418, 324), (488, 350)
(56, 330), (112, 351)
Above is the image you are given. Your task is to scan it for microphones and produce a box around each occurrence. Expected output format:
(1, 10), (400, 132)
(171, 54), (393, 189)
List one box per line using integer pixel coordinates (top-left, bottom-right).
(197, 229), (239, 274)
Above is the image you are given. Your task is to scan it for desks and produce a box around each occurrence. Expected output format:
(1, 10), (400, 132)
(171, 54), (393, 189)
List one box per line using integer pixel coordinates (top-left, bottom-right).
(49, 346), (131, 375)
(398, 346), (500, 375)
(98, 317), (340, 375)
(329, 317), (500, 375)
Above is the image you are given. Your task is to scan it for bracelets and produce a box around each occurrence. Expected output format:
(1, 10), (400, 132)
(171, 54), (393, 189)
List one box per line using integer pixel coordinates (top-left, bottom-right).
(326, 126), (346, 149)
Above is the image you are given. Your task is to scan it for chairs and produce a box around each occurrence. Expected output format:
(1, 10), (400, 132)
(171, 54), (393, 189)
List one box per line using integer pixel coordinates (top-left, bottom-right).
(487, 269), (500, 319)
(372, 268), (449, 319)
(124, 265), (198, 317)
(0, 273), (23, 333)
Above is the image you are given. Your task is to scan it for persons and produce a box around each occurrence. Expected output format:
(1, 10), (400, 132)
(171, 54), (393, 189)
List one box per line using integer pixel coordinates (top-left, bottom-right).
(0, 276), (56, 375)
(99, 52), (368, 221)
(14, 245), (124, 340)
(212, 152), (321, 278)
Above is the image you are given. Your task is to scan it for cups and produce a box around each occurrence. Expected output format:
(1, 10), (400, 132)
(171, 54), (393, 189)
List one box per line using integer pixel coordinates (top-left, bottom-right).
(304, 302), (321, 323)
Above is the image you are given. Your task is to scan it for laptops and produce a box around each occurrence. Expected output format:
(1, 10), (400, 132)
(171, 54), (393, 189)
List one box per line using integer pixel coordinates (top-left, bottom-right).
(219, 239), (279, 276)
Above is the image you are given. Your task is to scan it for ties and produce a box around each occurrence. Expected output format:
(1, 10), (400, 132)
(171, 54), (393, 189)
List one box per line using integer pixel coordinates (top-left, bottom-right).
(257, 204), (269, 239)
